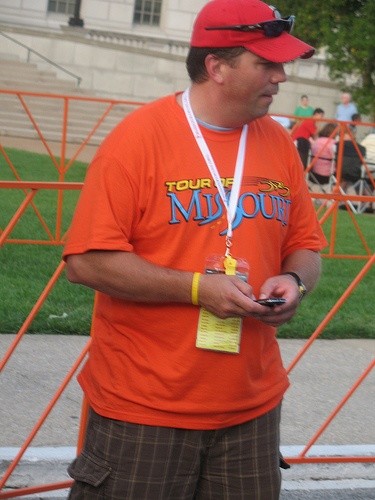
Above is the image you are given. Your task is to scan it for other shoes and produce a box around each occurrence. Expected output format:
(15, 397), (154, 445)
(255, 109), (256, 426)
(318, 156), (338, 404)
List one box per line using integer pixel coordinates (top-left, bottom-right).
(365, 207), (374, 213)
(340, 204), (357, 210)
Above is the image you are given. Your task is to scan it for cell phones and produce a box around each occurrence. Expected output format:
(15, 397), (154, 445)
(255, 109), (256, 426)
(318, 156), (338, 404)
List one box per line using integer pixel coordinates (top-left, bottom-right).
(253, 297), (287, 306)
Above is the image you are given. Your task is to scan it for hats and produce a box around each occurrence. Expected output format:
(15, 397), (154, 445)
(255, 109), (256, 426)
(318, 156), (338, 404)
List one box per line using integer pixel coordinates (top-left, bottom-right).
(190, 0), (315, 64)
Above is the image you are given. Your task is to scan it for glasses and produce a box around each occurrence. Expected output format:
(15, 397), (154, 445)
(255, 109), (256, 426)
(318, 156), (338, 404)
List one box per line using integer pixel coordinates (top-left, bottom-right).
(204, 15), (296, 37)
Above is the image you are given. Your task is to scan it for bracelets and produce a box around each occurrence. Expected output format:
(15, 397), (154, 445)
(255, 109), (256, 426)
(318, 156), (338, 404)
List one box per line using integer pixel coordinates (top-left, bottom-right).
(192, 272), (201, 305)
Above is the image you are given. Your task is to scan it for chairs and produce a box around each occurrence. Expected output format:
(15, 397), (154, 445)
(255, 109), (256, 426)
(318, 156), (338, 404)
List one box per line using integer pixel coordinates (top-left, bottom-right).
(294, 136), (334, 208)
(326, 140), (375, 214)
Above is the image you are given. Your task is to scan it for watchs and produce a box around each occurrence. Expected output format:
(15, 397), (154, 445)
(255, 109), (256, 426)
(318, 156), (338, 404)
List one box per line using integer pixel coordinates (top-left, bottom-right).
(283, 272), (307, 302)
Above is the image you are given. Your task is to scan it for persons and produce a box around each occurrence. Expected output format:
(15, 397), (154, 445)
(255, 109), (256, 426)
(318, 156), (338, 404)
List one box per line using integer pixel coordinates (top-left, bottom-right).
(63, 0), (328, 500)
(290, 92), (375, 186)
